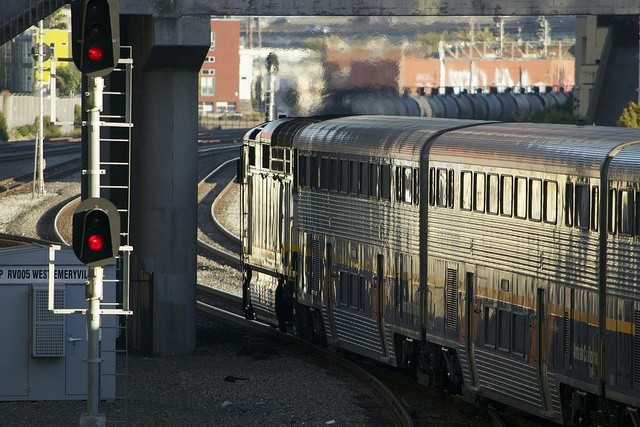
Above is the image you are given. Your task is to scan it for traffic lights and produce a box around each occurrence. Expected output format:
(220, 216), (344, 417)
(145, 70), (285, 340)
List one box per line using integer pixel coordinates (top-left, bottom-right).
(73, 198), (120, 267)
(71, 1), (120, 78)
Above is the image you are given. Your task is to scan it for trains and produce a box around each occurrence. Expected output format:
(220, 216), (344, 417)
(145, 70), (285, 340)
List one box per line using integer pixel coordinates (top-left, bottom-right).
(237, 116), (640, 427)
(325, 85), (573, 120)
